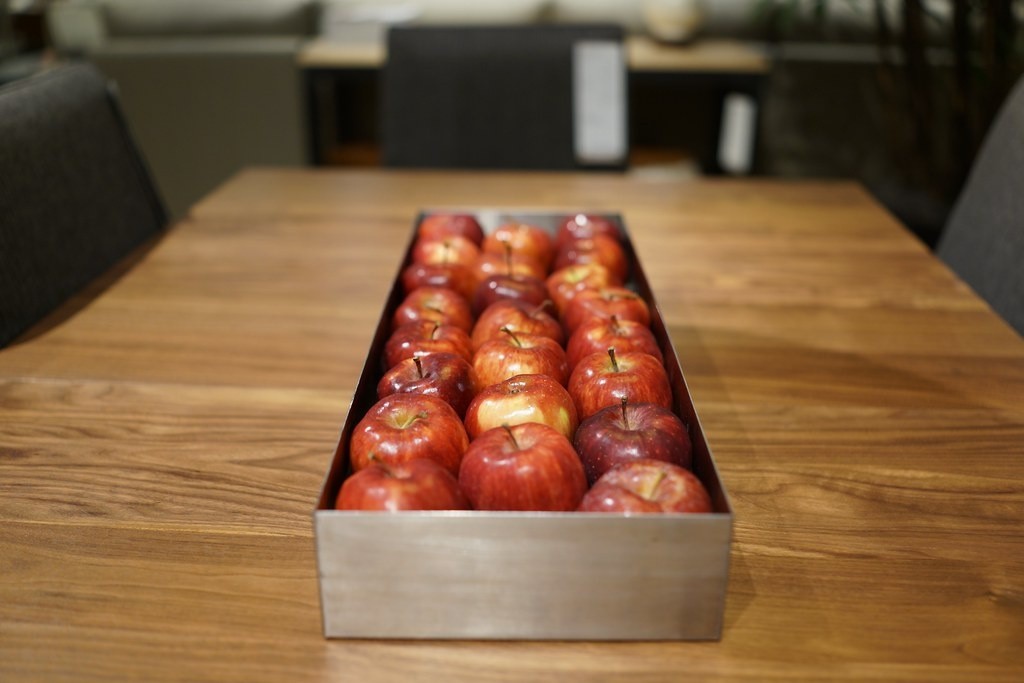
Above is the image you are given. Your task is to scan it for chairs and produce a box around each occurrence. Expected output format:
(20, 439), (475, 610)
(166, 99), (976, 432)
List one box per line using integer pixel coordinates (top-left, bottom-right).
(929, 79), (1024, 348)
(2, 48), (170, 352)
(385, 20), (633, 174)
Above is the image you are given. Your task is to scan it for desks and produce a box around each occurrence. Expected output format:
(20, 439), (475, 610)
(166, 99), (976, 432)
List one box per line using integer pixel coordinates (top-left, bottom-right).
(294, 21), (772, 178)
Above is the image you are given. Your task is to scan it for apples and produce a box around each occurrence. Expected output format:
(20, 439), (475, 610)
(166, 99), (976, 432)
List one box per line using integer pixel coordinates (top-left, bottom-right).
(335, 216), (715, 514)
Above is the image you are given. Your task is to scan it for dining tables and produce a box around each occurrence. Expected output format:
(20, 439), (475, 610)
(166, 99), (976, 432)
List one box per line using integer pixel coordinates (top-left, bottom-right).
(1, 165), (1024, 681)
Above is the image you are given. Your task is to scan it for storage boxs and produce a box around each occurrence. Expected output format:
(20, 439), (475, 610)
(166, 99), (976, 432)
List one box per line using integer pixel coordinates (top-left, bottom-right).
(309, 203), (742, 644)
